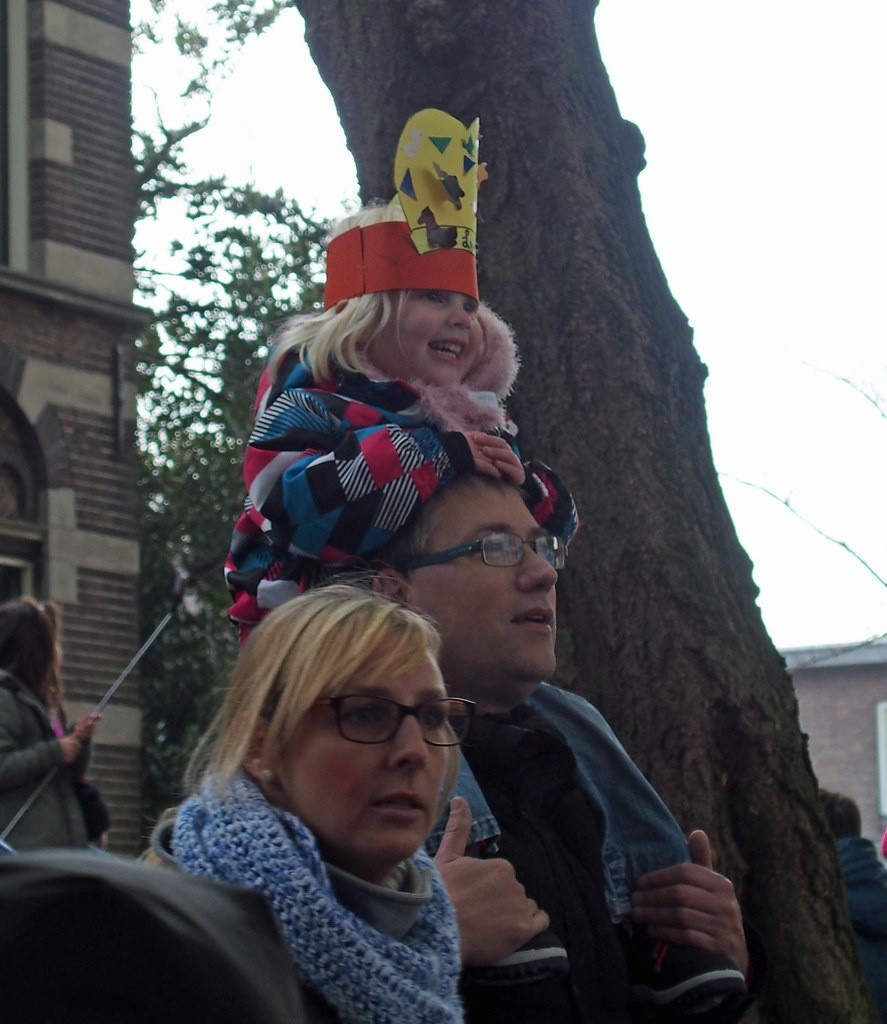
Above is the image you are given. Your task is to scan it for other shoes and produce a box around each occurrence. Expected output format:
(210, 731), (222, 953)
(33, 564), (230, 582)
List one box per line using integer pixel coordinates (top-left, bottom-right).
(626, 928), (745, 1018)
(462, 926), (569, 988)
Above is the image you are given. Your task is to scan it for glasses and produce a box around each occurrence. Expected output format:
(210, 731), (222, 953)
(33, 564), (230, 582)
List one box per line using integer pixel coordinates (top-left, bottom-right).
(396, 535), (569, 573)
(316, 692), (479, 747)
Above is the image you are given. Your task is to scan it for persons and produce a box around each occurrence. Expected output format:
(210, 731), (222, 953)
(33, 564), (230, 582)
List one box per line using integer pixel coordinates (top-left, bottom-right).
(220, 189), (751, 1024)
(0, 850), (308, 1023)
(819, 787), (887, 1024)
(0, 596), (103, 851)
(138, 580), (464, 1024)
(75, 778), (110, 853)
